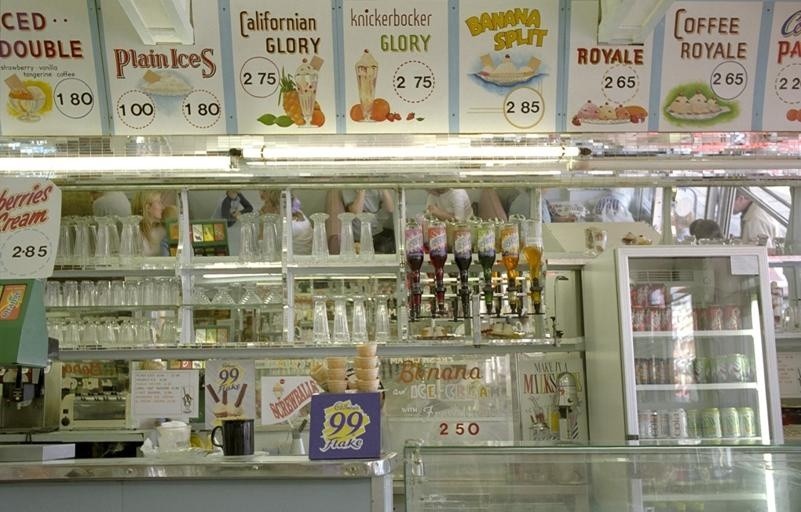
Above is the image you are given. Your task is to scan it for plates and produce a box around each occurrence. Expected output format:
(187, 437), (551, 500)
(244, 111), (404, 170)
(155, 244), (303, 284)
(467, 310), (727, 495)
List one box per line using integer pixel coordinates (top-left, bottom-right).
(577, 117), (630, 125)
(664, 104), (733, 120)
(138, 85), (193, 100)
(207, 451), (269, 463)
(477, 70), (544, 88)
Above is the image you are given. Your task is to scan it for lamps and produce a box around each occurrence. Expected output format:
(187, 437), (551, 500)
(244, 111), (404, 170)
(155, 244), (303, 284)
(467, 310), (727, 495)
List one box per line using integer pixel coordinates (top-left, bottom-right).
(234, 143), (582, 166)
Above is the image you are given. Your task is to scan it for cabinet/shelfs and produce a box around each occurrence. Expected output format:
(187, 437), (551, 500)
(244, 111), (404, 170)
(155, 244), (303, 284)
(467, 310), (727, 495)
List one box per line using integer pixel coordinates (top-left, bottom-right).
(0, 183), (801, 512)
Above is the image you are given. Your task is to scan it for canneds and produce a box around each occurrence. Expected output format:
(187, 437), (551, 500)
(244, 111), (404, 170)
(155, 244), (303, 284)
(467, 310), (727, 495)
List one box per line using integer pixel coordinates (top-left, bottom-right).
(630, 283), (758, 445)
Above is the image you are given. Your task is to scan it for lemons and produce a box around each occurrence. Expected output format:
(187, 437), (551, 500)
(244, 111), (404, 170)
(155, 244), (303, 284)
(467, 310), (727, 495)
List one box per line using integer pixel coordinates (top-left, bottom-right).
(276, 116), (294, 126)
(257, 114), (275, 125)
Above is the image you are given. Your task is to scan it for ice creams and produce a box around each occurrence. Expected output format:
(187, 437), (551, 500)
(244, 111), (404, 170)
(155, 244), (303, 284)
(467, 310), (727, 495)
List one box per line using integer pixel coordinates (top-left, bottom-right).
(354, 49), (377, 85)
(294, 58), (318, 94)
(227, 384), (247, 416)
(272, 383), (284, 398)
(207, 384), (227, 418)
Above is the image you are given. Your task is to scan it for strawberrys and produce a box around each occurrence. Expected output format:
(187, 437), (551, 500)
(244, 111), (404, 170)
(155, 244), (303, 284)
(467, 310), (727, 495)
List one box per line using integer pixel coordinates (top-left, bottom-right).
(387, 112), (414, 122)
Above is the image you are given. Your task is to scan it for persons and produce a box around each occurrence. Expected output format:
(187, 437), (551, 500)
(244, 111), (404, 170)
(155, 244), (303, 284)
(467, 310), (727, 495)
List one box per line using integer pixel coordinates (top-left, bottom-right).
(86, 186), (793, 301)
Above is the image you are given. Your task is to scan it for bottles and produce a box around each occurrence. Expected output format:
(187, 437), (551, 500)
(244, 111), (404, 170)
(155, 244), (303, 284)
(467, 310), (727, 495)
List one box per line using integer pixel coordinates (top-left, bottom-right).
(451, 222), (473, 321)
(474, 220), (496, 314)
(500, 221), (520, 313)
(519, 217), (543, 308)
(287, 430), (305, 455)
(401, 219), (424, 310)
(427, 218), (448, 308)
(553, 371), (578, 447)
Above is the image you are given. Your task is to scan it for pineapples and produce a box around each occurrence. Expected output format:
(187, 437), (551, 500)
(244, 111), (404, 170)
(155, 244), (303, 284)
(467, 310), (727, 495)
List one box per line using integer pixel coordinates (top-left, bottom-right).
(283, 90), (319, 124)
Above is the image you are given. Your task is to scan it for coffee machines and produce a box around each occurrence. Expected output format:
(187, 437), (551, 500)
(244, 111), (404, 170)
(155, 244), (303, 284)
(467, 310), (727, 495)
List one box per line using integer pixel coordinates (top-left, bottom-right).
(3, 360), (63, 431)
(65, 359), (128, 418)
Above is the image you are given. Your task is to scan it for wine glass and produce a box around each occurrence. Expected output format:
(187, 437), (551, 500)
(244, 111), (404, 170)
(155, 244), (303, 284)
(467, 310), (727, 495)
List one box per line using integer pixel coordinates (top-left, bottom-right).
(7, 96), (46, 122)
(771, 279), (801, 333)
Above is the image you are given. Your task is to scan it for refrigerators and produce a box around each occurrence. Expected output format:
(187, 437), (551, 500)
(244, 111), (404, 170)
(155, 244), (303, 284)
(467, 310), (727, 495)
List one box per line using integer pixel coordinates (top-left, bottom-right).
(592, 452), (787, 512)
(578, 243), (786, 446)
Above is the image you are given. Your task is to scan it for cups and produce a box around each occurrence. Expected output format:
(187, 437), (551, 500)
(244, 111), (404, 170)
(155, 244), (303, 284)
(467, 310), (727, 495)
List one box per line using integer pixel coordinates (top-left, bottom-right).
(303, 208), (380, 267)
(41, 278), (177, 308)
(187, 282), (284, 313)
(210, 418), (256, 454)
(581, 225), (785, 258)
(307, 293), (392, 345)
(50, 317), (176, 351)
(354, 64), (381, 124)
(59, 212), (146, 272)
(235, 214), (280, 265)
(154, 425), (191, 455)
(293, 73), (319, 131)
